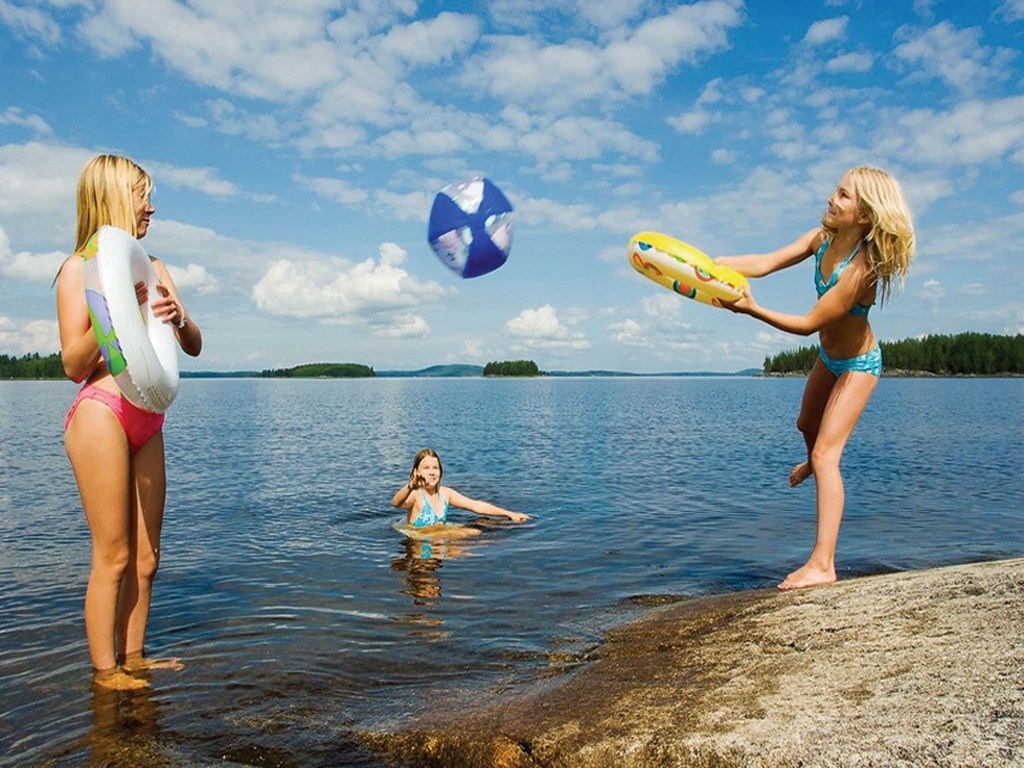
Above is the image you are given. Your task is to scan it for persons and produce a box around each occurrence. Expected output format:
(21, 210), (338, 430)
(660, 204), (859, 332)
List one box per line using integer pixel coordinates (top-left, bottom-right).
(392, 448), (532, 528)
(51, 154), (202, 690)
(712, 165), (916, 592)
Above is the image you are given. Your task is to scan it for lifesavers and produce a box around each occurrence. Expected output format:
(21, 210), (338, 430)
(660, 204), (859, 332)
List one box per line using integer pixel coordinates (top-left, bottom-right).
(82, 220), (180, 415)
(627, 231), (752, 315)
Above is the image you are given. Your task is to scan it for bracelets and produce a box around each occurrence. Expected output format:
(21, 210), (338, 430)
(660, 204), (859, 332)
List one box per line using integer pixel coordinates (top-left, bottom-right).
(175, 303), (187, 327)
(407, 483), (412, 490)
